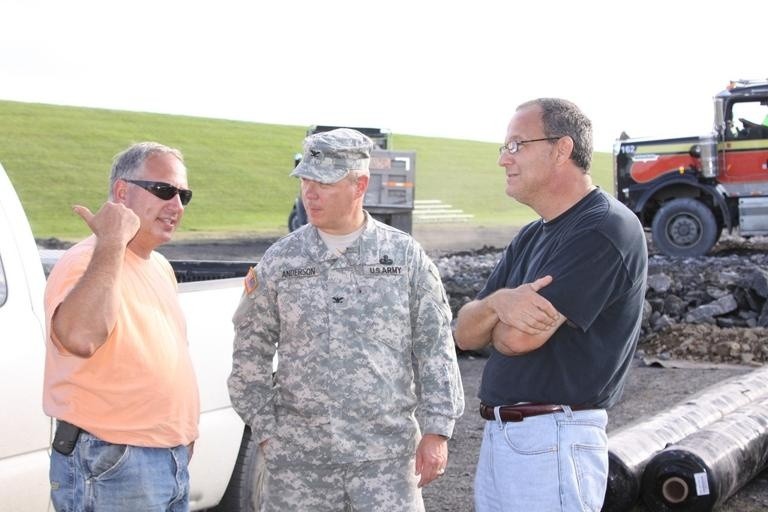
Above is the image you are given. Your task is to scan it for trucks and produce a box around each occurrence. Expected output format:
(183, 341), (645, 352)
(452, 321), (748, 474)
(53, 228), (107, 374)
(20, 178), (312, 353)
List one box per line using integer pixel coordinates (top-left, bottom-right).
(289, 126), (416, 240)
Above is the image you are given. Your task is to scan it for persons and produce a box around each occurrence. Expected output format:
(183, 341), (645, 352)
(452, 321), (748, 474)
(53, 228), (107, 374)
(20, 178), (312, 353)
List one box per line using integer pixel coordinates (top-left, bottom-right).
(225, 128), (466, 511)
(454, 98), (648, 511)
(42, 142), (199, 512)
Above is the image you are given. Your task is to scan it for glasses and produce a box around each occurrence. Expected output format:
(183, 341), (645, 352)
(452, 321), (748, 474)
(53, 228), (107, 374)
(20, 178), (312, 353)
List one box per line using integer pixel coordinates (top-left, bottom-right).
(499, 135), (560, 158)
(123, 178), (192, 206)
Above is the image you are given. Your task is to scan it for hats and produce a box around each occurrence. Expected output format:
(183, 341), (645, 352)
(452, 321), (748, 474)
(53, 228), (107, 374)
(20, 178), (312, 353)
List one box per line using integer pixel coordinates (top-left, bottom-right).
(288, 128), (374, 184)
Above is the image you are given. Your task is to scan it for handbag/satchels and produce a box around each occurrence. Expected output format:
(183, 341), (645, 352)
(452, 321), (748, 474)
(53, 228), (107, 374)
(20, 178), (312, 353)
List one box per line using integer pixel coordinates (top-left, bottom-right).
(53, 422), (80, 454)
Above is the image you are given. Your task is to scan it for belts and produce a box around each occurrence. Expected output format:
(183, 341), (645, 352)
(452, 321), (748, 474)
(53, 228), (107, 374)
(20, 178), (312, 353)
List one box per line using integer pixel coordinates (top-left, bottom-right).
(480, 403), (600, 422)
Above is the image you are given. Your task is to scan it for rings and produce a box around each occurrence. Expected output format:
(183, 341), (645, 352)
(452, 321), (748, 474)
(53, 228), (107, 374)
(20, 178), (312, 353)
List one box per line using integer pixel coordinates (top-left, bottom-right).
(437, 468), (445, 476)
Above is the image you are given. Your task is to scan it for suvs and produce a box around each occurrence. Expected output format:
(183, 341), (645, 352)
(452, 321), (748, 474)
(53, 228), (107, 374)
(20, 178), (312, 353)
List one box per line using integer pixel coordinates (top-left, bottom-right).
(614, 79), (768, 256)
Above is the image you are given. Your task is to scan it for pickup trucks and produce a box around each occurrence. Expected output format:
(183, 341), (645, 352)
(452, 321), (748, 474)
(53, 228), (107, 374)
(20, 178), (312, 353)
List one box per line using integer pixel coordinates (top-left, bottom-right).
(0, 158), (278, 512)
